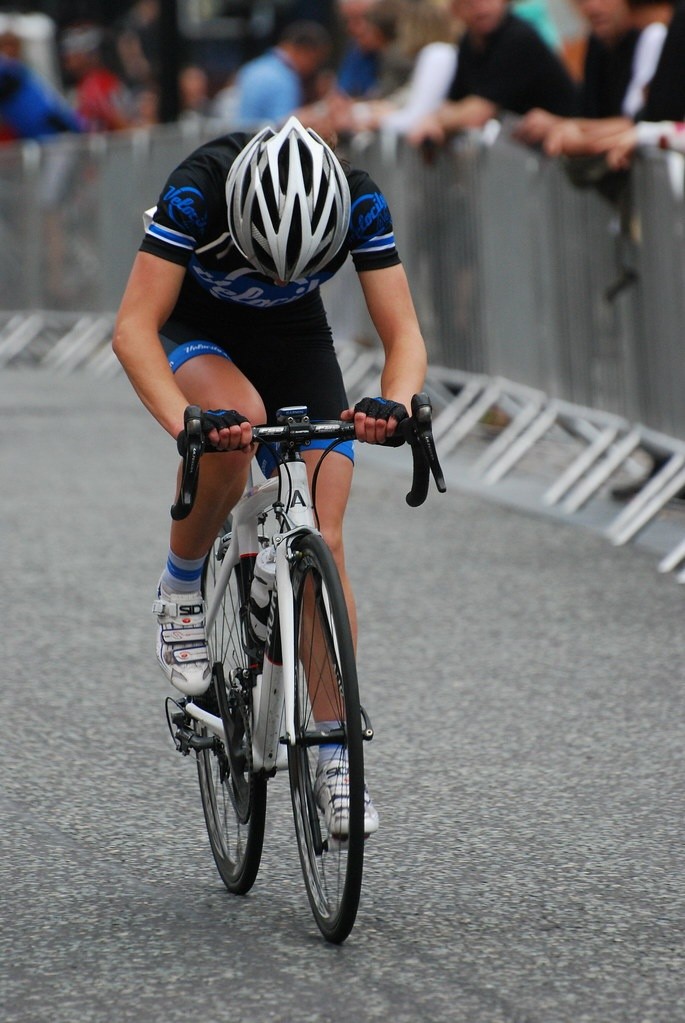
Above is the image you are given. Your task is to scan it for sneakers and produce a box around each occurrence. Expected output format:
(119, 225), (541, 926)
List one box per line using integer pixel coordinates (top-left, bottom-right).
(152, 573), (213, 694)
(313, 759), (379, 838)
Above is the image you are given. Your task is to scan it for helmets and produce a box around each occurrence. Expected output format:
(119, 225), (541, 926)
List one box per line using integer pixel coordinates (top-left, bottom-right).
(225, 113), (350, 289)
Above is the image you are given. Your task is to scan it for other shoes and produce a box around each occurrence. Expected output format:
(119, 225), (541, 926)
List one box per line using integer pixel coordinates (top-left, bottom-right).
(478, 405), (510, 439)
(610, 461), (685, 503)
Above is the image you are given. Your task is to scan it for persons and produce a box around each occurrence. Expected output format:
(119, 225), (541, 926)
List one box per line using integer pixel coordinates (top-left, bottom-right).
(112, 117), (427, 834)
(0, 0), (685, 505)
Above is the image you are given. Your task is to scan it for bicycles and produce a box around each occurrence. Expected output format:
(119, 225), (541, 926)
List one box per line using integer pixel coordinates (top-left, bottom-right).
(165, 392), (448, 947)
